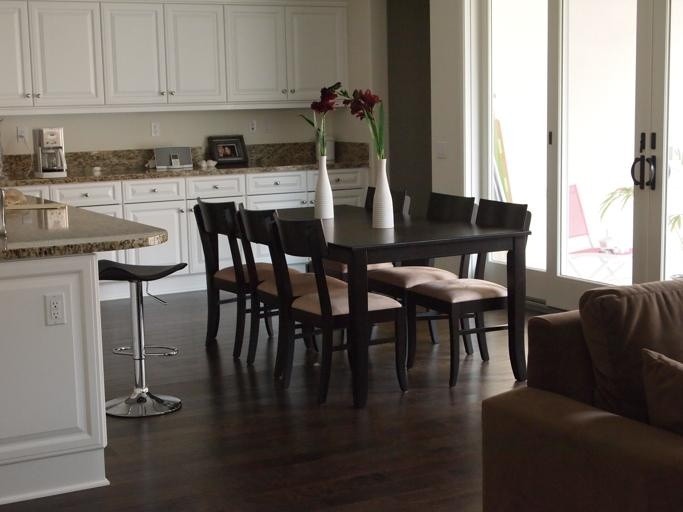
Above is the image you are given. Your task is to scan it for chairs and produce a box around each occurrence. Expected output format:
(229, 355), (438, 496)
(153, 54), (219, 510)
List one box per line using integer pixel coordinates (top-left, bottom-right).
(478, 309), (682, 511)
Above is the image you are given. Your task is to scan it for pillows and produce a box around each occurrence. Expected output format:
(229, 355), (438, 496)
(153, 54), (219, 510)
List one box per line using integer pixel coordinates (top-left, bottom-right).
(578, 279), (682, 429)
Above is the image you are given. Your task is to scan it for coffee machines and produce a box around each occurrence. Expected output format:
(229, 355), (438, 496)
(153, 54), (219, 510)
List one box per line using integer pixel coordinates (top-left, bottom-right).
(31, 127), (67, 178)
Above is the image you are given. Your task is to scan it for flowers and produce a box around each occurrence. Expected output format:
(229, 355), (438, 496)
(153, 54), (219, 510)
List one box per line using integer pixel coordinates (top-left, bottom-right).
(301, 81), (341, 158)
(336, 85), (387, 162)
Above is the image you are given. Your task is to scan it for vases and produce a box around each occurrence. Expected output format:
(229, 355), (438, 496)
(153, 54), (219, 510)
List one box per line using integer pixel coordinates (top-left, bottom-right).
(313, 155), (336, 221)
(370, 158), (396, 232)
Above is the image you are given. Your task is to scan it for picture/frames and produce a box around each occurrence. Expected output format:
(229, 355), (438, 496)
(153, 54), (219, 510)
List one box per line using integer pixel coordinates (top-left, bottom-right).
(207, 135), (249, 167)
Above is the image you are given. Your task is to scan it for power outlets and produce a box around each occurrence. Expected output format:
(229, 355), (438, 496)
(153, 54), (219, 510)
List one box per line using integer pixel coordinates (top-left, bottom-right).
(17, 127), (24, 142)
(150, 121), (159, 138)
(249, 120), (257, 134)
